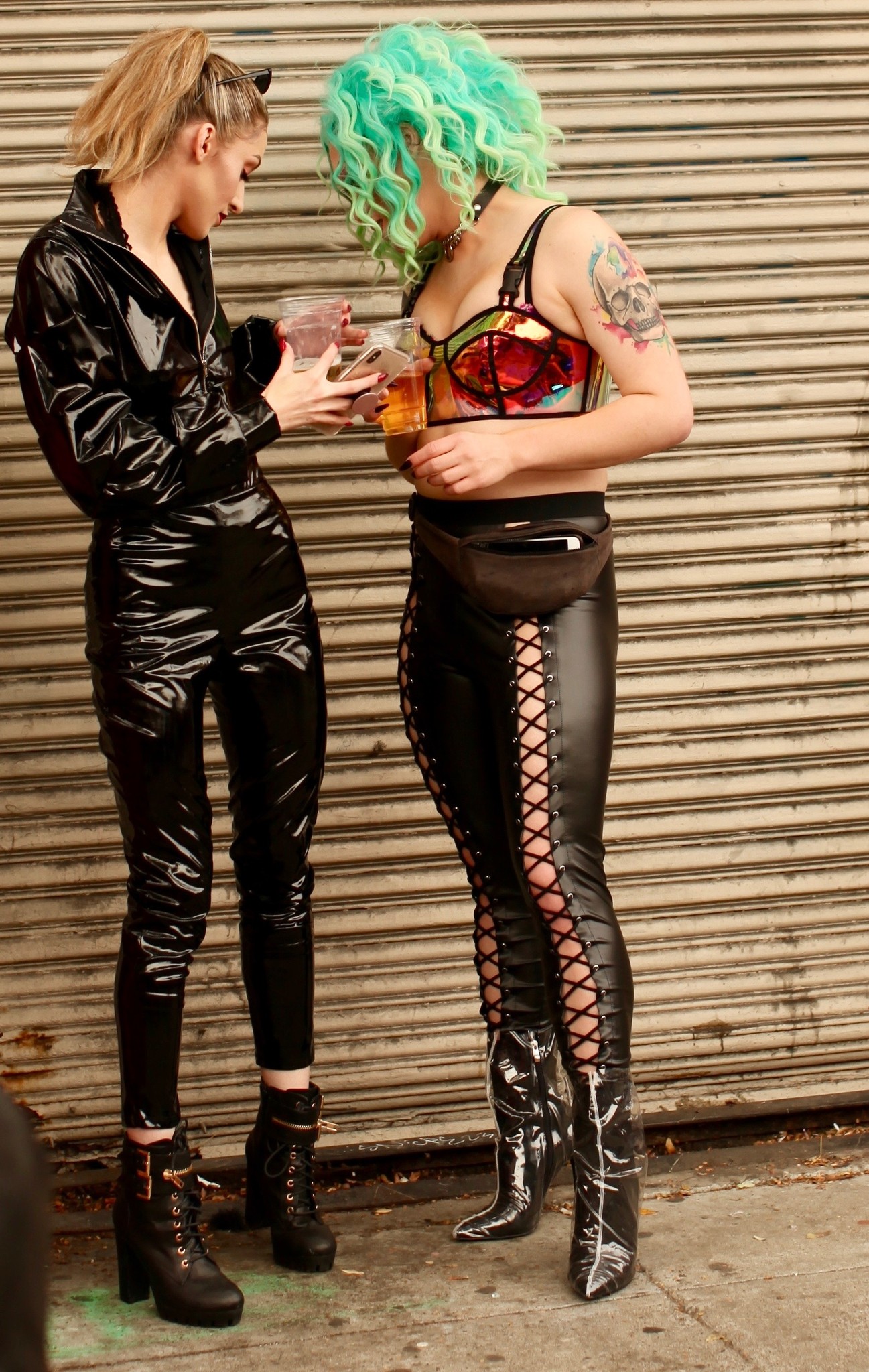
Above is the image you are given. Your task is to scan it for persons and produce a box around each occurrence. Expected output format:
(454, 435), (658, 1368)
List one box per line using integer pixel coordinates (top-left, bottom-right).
(314, 17), (695, 1301)
(4, 23), (388, 1329)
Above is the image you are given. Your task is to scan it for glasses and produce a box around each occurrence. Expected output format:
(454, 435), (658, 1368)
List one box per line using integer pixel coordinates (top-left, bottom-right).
(192, 68), (274, 107)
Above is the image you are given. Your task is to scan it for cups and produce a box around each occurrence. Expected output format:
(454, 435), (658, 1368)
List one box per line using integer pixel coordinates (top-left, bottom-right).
(275, 294), (346, 383)
(357, 316), (429, 438)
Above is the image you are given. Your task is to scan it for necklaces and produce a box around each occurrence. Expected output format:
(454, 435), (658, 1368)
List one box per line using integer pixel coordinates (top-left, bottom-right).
(439, 178), (504, 263)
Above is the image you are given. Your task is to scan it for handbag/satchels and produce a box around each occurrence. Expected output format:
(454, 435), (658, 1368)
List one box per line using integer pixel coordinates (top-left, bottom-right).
(411, 511), (615, 617)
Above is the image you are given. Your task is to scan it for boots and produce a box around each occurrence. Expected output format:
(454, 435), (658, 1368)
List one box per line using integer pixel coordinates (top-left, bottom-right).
(245, 1080), (338, 1273)
(569, 1066), (641, 1301)
(451, 1021), (576, 1243)
(112, 1118), (244, 1329)
